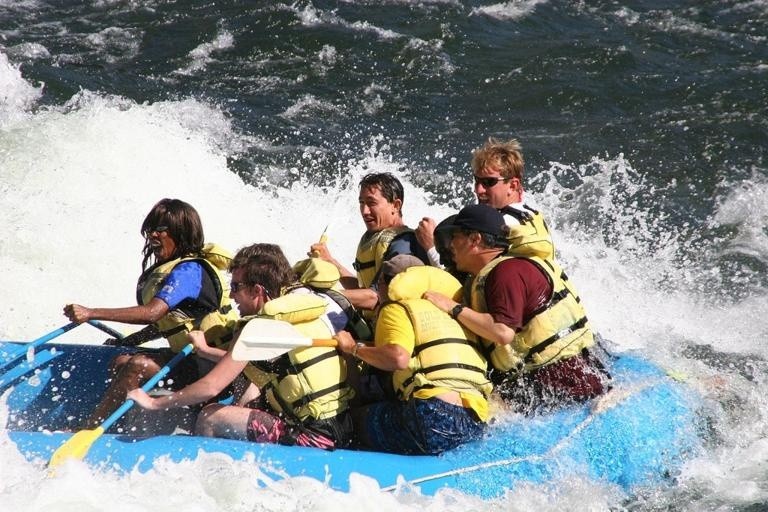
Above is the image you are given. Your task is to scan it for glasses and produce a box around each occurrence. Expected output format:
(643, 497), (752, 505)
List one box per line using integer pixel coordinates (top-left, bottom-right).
(474, 175), (514, 188)
(145, 225), (171, 235)
(230, 281), (255, 292)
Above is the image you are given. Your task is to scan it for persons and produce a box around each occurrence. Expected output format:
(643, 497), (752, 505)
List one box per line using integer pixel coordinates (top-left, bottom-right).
(63, 198), (238, 431)
(420, 204), (612, 412)
(127, 243), (361, 451)
(415, 140), (553, 268)
(310, 172), (427, 320)
(334, 254), (493, 455)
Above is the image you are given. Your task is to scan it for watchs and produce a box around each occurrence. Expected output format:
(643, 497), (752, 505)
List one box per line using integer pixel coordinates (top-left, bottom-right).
(352, 342), (367, 357)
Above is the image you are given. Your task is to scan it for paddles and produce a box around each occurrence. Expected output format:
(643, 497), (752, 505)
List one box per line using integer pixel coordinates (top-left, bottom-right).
(48, 342), (197, 477)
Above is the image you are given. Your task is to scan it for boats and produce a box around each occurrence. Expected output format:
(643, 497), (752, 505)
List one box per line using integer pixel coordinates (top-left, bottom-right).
(0, 340), (709, 504)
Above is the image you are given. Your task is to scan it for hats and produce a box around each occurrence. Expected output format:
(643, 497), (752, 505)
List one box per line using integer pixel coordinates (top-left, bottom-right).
(381, 252), (426, 278)
(436, 203), (510, 238)
